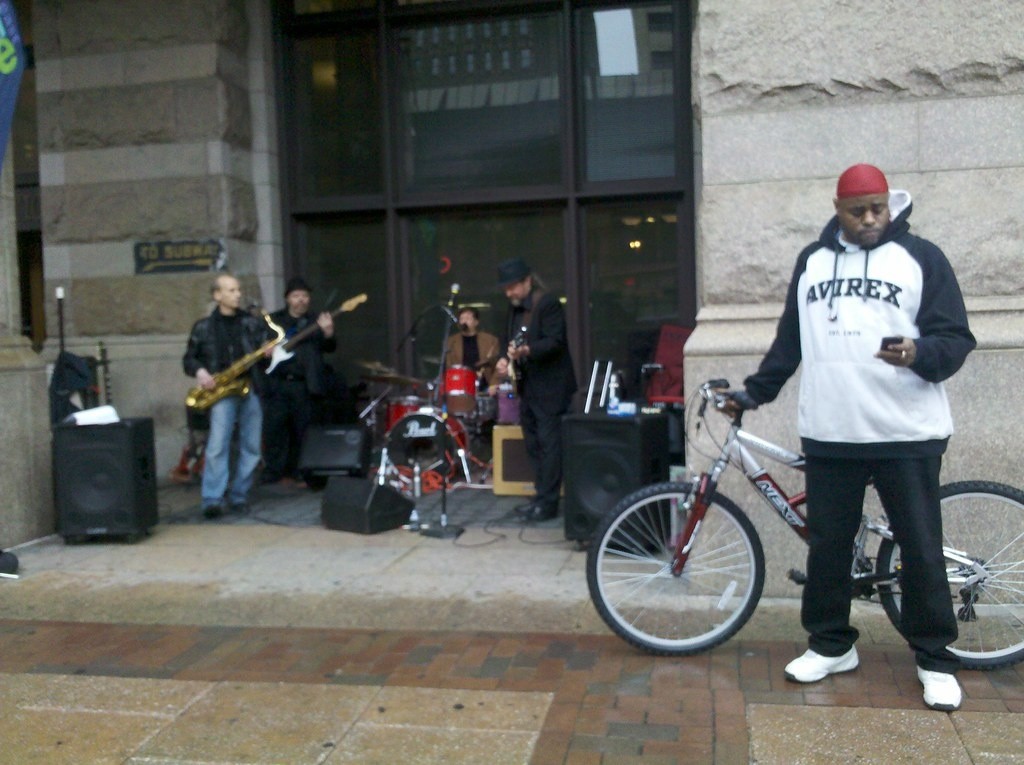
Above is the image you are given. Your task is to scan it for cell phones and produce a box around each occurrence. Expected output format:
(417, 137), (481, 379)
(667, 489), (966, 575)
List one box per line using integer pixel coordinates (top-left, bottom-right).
(880, 337), (902, 352)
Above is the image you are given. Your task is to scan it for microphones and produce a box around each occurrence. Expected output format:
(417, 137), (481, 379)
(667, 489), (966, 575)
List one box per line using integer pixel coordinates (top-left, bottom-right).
(446, 283), (460, 308)
(460, 322), (468, 331)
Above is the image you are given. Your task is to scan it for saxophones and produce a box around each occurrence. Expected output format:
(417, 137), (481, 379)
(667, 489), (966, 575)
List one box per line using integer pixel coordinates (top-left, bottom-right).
(184, 299), (286, 411)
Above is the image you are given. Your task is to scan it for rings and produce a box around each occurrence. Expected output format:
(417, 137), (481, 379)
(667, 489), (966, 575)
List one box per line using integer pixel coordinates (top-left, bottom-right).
(901, 350), (907, 359)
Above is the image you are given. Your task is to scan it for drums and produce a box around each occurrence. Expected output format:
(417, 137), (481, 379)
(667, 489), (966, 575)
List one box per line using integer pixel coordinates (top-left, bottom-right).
(386, 406), (469, 484)
(385, 394), (427, 439)
(439, 363), (478, 417)
(463, 396), (496, 423)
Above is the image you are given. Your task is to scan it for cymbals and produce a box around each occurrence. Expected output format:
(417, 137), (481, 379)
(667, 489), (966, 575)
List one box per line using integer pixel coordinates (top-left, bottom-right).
(362, 372), (422, 385)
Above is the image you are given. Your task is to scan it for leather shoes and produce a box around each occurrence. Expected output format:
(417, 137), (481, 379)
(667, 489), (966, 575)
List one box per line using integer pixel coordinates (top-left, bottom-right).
(527, 505), (552, 521)
(515, 500), (535, 513)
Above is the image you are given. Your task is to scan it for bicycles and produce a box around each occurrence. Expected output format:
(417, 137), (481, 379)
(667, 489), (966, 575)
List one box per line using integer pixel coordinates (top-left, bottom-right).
(584, 377), (1024, 672)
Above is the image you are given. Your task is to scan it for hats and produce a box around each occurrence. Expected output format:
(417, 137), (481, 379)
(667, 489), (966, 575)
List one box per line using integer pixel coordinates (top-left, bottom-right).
(836, 162), (888, 199)
(497, 258), (530, 286)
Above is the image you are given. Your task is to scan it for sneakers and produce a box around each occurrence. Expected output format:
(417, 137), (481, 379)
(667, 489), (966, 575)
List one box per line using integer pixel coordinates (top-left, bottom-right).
(917, 663), (962, 710)
(785, 642), (859, 682)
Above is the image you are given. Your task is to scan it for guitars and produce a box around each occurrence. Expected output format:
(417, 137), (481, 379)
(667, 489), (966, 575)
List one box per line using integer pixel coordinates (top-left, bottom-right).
(96, 340), (113, 405)
(264, 293), (368, 376)
(506, 325), (527, 398)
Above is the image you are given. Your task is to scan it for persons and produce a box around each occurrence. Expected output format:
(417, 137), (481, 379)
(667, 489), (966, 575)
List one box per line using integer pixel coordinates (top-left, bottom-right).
(710, 164), (979, 714)
(183, 275), (275, 518)
(260, 277), (338, 497)
(444, 304), (501, 444)
(496, 256), (576, 522)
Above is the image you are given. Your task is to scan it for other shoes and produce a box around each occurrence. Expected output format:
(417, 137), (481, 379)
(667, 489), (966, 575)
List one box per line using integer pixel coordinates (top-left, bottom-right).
(278, 477), (293, 489)
(293, 476), (306, 488)
(229, 501), (251, 515)
(202, 505), (222, 519)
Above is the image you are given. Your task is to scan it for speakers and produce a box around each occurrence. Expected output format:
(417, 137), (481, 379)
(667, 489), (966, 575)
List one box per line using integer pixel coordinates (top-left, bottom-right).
(53, 417), (158, 545)
(562, 408), (670, 555)
(493, 425), (566, 496)
(298, 424), (415, 534)
